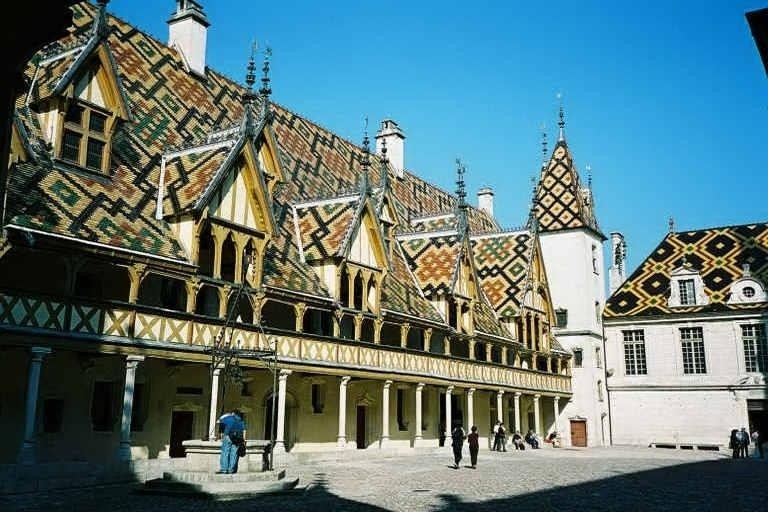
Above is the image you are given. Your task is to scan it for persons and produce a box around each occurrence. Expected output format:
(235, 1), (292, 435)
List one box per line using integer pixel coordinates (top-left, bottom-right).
(549, 431), (559, 448)
(492, 421), (501, 451)
(216, 410), (247, 473)
(729, 427), (764, 459)
(497, 422), (507, 452)
(525, 429), (538, 448)
(451, 420), (467, 469)
(467, 426), (479, 469)
(512, 430), (524, 450)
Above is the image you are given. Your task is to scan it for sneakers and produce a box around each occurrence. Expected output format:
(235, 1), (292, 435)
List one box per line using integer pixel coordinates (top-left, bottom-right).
(215, 469), (237, 474)
(493, 446), (540, 452)
(455, 465), (476, 469)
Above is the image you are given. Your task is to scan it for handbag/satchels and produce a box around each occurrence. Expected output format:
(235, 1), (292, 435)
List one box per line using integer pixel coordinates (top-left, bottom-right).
(229, 430), (244, 446)
(237, 444), (246, 457)
